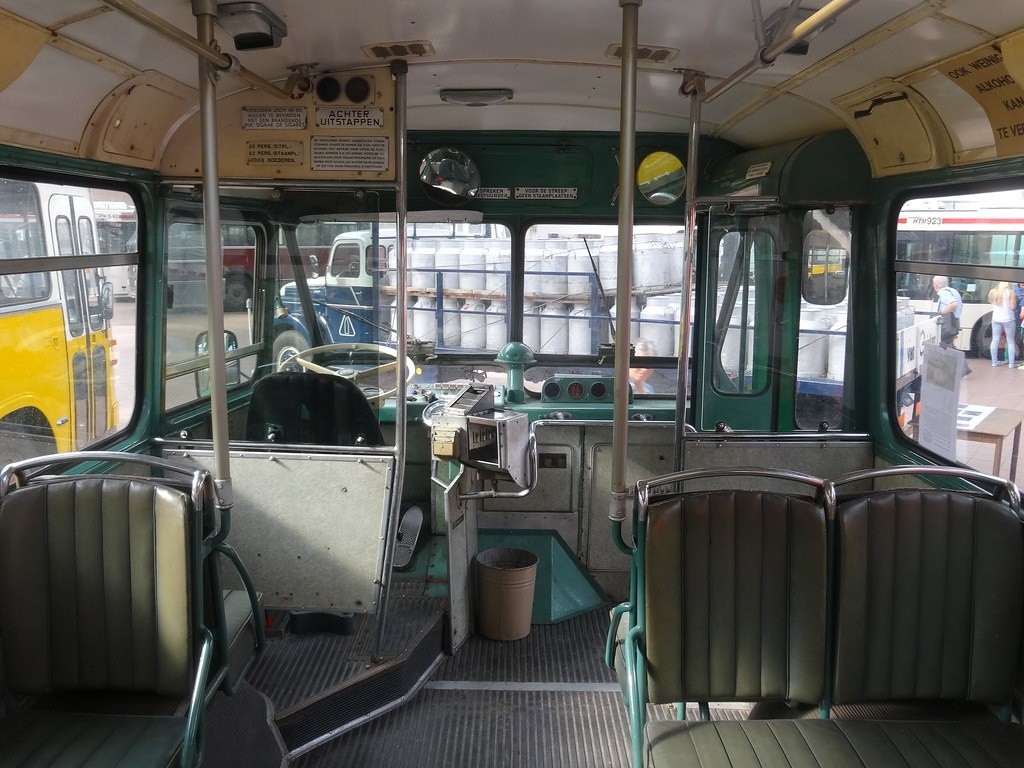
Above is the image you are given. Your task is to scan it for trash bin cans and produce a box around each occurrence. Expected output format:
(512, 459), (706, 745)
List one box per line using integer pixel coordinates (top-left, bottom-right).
(476, 547), (540, 642)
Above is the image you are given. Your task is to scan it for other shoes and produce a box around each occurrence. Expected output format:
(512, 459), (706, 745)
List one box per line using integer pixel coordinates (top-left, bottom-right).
(962, 371), (973, 379)
(992, 362), (1001, 366)
(1008, 364), (1017, 368)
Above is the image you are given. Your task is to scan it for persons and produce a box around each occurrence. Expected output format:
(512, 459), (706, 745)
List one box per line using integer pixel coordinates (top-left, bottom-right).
(1014, 282), (1024, 371)
(628, 338), (658, 393)
(932, 276), (972, 378)
(987, 281), (1019, 368)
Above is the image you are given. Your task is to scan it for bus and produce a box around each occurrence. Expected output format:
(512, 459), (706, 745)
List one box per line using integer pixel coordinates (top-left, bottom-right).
(0, 180), (121, 474)
(0, 200), (365, 316)
(803, 193), (1024, 363)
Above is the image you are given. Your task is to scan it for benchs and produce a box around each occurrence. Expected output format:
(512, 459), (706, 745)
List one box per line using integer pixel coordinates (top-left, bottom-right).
(602, 465), (1023, 768)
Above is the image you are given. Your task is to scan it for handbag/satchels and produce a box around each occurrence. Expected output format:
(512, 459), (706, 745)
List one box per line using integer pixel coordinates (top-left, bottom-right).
(937, 311), (960, 339)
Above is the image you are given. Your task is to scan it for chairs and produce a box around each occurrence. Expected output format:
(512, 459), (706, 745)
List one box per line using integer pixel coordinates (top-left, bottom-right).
(241, 371), (385, 447)
(0, 450), (212, 768)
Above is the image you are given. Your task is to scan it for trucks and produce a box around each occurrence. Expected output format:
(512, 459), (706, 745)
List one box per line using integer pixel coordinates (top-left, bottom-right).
(247, 226), (924, 444)
